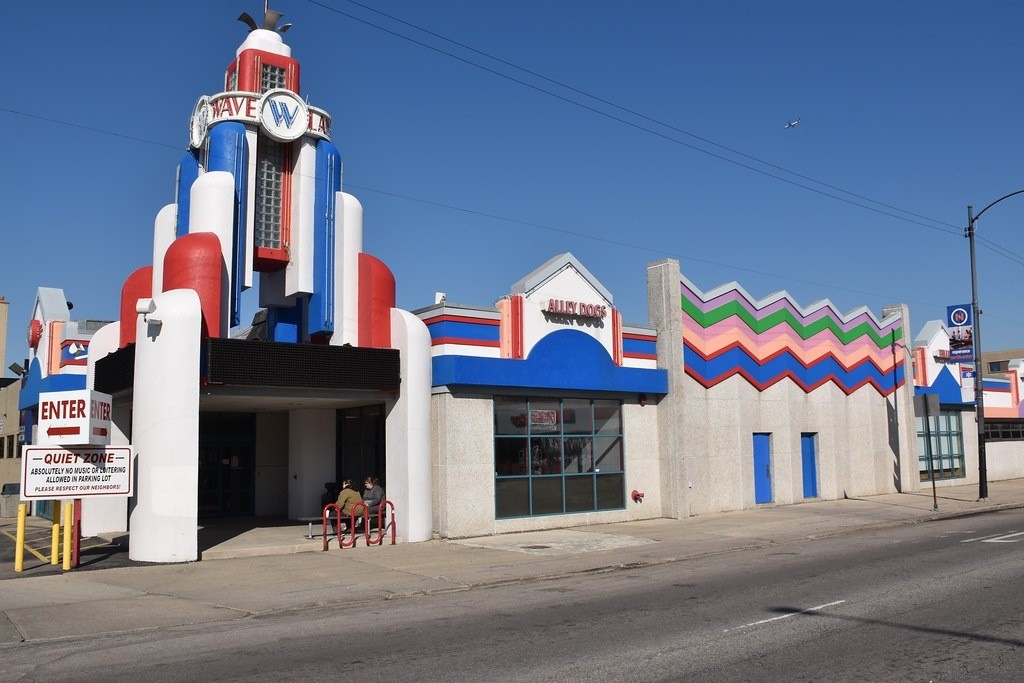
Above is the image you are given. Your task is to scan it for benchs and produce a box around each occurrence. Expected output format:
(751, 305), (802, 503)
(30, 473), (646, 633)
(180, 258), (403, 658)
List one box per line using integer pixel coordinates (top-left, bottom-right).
(297, 511), (387, 539)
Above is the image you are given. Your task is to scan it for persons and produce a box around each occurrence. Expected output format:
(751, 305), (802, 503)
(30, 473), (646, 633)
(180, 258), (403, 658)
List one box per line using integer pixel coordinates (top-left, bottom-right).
(362, 477), (387, 514)
(329, 480), (364, 533)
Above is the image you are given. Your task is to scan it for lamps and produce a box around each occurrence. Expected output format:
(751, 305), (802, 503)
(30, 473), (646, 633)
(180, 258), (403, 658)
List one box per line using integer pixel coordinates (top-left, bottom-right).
(68, 342), (88, 360)
(135, 298), (162, 326)
